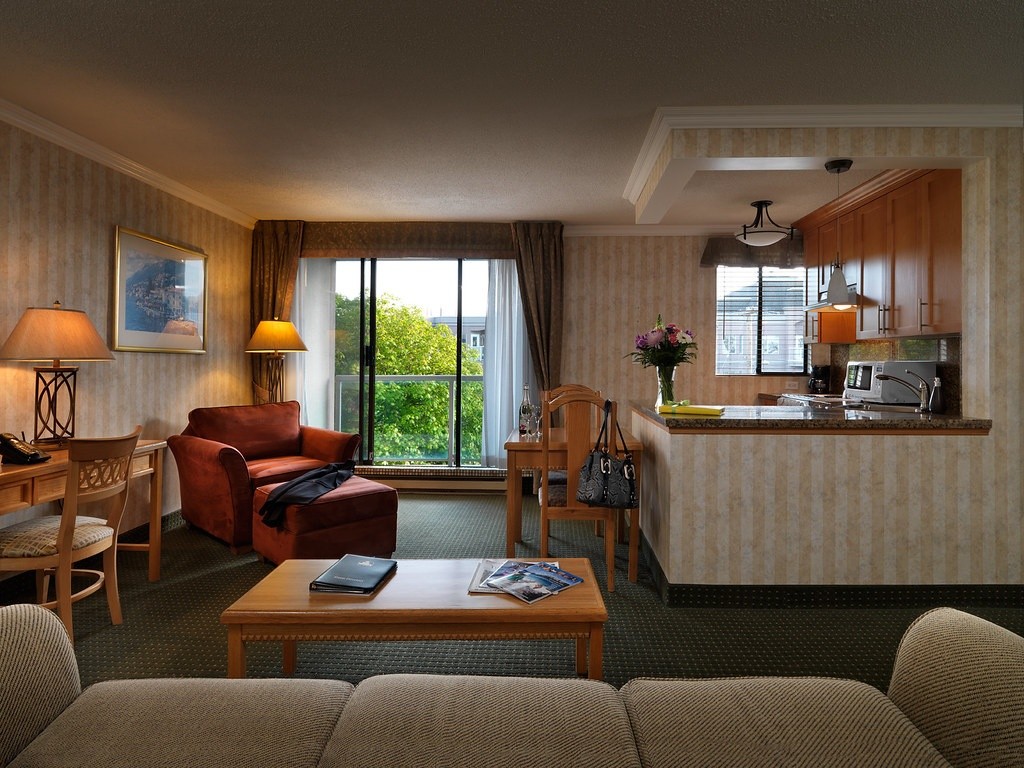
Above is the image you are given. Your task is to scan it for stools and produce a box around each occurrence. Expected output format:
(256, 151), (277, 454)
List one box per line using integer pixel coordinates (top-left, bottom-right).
(253, 473), (398, 564)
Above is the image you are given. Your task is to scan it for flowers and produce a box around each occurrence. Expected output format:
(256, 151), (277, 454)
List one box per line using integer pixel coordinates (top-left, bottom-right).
(622, 313), (699, 403)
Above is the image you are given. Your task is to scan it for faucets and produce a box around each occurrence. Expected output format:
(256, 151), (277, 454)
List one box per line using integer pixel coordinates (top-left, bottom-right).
(876, 369), (931, 414)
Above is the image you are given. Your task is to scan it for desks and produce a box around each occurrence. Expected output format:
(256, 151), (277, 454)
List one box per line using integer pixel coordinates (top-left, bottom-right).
(505, 427), (643, 581)
(0, 439), (167, 582)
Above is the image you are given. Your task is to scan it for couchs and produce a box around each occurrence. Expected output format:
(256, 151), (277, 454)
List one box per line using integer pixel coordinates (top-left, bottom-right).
(0, 605), (1024, 768)
(168, 399), (362, 555)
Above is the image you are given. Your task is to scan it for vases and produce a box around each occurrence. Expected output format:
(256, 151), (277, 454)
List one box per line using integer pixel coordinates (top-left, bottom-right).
(655, 364), (675, 406)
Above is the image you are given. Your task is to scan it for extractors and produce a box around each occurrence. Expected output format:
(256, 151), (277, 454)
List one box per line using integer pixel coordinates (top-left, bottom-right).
(803, 284), (857, 313)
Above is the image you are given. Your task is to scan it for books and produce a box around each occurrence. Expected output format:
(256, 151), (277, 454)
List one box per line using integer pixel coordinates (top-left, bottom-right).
(468, 559), (585, 605)
(310, 553), (398, 596)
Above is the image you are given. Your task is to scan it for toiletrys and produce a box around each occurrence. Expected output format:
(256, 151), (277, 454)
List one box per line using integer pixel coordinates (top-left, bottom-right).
(928, 376), (947, 412)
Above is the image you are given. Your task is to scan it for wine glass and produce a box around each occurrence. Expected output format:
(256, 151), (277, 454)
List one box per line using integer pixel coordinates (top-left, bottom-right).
(521, 405), (542, 438)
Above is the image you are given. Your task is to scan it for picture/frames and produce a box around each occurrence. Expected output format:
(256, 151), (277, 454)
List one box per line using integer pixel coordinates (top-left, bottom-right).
(113, 226), (210, 354)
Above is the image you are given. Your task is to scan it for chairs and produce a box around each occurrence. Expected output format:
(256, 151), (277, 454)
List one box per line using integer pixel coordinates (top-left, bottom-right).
(1, 424), (143, 647)
(539, 383), (618, 593)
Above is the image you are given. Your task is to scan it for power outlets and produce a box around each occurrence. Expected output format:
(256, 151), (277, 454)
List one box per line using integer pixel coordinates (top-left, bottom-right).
(784, 382), (798, 389)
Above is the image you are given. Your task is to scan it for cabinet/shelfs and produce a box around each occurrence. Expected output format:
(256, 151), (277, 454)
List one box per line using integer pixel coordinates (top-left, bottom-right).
(800, 169), (963, 345)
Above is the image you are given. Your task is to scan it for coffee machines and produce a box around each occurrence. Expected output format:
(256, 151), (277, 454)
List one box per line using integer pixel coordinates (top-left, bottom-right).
(808, 365), (831, 394)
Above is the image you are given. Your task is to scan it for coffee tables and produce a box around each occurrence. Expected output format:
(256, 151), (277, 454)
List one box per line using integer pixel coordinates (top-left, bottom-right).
(220, 557), (608, 681)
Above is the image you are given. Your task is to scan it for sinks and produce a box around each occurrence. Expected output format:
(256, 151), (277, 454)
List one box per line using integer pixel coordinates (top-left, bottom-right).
(841, 403), (917, 415)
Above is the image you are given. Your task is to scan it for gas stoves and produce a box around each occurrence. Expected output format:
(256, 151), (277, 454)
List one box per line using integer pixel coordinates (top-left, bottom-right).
(776, 394), (842, 406)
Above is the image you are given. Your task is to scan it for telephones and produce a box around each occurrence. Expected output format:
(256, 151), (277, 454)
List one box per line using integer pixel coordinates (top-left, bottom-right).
(0, 431), (51, 465)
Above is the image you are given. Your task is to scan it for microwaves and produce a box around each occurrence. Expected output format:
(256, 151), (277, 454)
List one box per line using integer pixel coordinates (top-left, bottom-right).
(844, 361), (936, 405)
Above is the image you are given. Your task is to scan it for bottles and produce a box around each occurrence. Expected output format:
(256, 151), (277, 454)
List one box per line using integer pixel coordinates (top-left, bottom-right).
(519, 384), (531, 433)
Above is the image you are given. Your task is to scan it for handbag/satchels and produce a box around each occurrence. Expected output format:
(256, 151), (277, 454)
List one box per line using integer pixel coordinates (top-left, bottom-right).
(577, 399), (640, 509)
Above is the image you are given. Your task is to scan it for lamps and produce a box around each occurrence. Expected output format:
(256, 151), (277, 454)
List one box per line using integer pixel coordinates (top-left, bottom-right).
(825, 158), (852, 302)
(0, 301), (115, 453)
(734, 200), (790, 247)
(245, 317), (309, 403)
